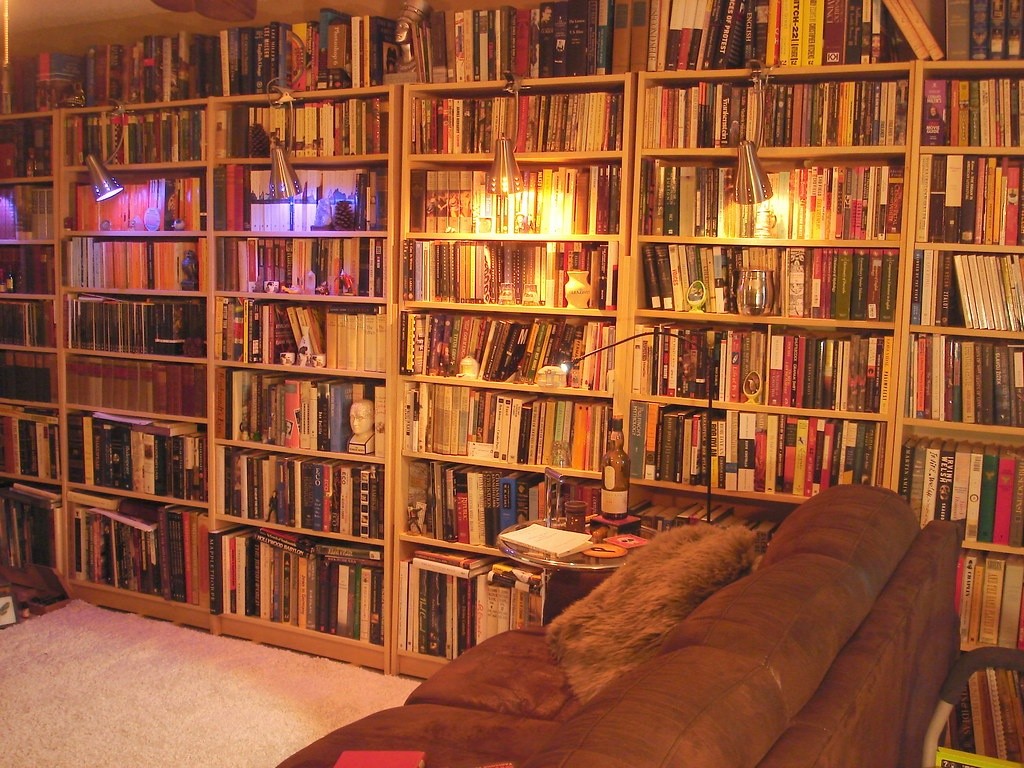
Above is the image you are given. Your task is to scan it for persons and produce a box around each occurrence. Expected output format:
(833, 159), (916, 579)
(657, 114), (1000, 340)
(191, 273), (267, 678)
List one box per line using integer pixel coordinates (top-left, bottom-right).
(405, 503), (423, 533)
(540, 6), (552, 24)
(265, 490), (279, 524)
(394, 21), (412, 62)
(928, 107), (939, 119)
(350, 399), (375, 444)
(557, 40), (564, 51)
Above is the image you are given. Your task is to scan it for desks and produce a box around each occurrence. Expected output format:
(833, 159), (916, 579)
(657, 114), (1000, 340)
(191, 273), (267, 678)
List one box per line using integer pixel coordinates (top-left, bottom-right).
(494, 513), (664, 629)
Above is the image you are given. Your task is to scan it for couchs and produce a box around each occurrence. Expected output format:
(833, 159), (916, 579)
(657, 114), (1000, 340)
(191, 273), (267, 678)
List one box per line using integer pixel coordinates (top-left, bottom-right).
(274, 481), (968, 768)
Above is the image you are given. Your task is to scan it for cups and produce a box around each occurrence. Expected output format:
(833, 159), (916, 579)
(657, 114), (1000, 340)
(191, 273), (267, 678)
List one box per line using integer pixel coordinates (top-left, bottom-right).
(280, 352), (295, 365)
(310, 354), (327, 368)
(736, 268), (775, 316)
(263, 280), (280, 294)
(565, 500), (589, 533)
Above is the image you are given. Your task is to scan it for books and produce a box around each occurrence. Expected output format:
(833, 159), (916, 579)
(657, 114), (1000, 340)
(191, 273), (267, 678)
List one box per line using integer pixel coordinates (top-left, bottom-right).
(0, 0), (1024, 768)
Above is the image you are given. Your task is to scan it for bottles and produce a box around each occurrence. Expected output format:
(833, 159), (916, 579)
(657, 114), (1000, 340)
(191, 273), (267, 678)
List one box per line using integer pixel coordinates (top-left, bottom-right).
(572, 366), (581, 388)
(26, 148), (39, 178)
(565, 271), (592, 310)
(305, 271), (315, 295)
(599, 415), (631, 520)
(297, 326), (311, 367)
(0, 264), (14, 293)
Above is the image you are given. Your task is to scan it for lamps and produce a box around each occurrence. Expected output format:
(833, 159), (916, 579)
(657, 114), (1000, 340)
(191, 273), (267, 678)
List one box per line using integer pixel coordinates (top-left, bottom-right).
(557, 332), (716, 524)
(395, 0), (433, 43)
(484, 70), (525, 196)
(733, 69), (774, 205)
(269, 85), (303, 201)
(84, 98), (127, 202)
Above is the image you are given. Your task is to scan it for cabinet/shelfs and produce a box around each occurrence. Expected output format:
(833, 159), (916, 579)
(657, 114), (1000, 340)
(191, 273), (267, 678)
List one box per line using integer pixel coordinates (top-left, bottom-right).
(0, 1), (1024, 764)
